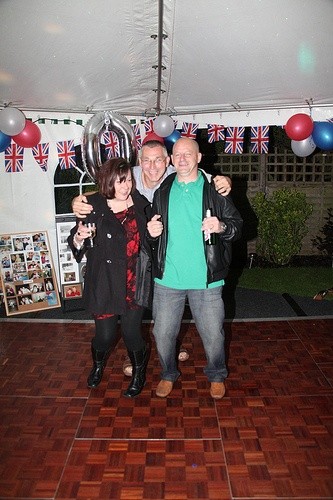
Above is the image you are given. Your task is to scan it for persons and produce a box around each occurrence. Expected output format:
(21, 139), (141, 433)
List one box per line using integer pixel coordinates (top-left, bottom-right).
(0, 225), (87, 311)
(67, 140), (243, 397)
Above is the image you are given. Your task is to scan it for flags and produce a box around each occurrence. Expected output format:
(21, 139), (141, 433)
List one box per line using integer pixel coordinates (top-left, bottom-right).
(5, 118), (333, 173)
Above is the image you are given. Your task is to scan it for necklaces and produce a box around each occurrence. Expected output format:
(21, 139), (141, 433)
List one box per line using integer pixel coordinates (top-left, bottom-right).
(107, 199), (128, 224)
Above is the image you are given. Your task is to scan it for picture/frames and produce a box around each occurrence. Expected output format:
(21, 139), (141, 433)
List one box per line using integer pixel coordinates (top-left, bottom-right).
(0, 231), (61, 316)
(62, 283), (82, 299)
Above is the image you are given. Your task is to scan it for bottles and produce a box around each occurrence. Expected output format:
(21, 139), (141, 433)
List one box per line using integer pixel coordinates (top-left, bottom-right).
(204, 208), (216, 245)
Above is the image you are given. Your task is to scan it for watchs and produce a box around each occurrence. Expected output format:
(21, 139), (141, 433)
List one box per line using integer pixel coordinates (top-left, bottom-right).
(220, 222), (227, 233)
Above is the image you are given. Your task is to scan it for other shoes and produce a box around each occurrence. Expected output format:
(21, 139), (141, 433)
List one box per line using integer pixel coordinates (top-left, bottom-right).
(209, 382), (226, 399)
(156, 379), (174, 398)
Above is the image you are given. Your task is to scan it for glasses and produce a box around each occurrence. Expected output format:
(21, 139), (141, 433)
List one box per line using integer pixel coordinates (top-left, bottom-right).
(143, 158), (166, 164)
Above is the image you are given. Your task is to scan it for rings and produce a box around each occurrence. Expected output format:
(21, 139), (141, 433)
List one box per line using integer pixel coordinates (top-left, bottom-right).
(77, 232), (81, 236)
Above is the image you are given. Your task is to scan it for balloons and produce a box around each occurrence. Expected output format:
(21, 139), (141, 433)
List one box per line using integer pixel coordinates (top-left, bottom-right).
(285, 114), (333, 157)
(143, 115), (182, 152)
(0, 108), (41, 153)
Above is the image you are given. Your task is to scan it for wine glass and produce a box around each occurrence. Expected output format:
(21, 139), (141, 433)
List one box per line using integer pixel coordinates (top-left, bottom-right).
(85, 223), (98, 248)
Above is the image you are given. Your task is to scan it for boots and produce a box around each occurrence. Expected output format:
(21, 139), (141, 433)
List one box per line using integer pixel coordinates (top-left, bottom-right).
(124, 349), (147, 396)
(87, 349), (106, 386)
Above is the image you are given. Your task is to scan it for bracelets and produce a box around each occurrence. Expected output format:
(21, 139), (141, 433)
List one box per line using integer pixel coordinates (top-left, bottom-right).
(73, 234), (84, 249)
